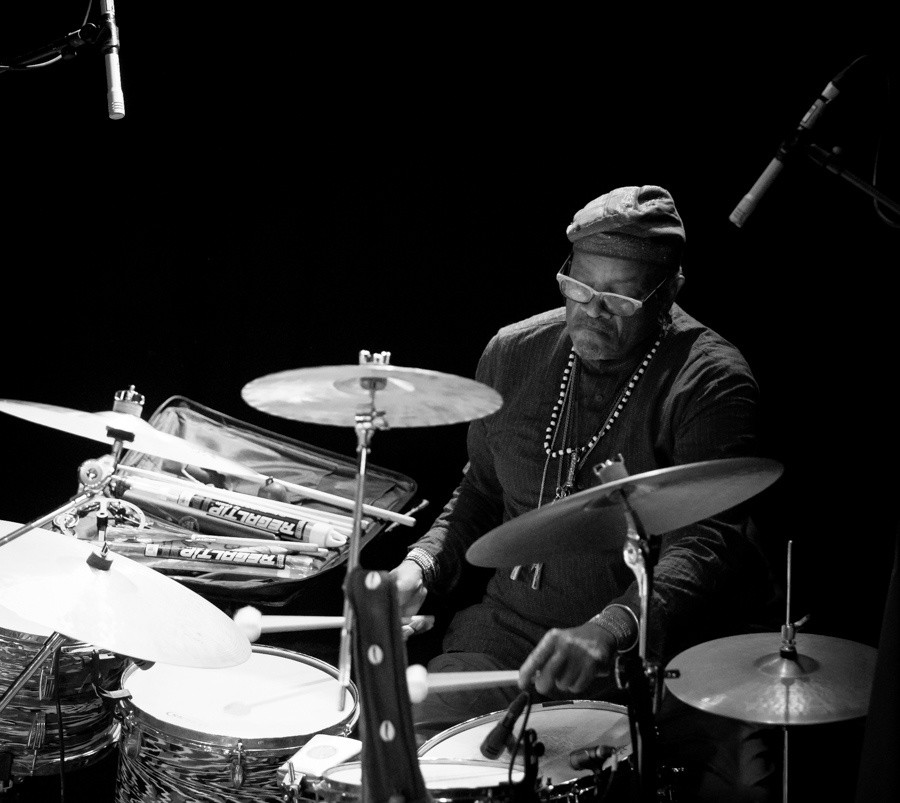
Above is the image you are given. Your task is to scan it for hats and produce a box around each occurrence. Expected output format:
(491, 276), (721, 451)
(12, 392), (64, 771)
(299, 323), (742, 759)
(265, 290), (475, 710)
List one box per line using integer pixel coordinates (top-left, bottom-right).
(567, 185), (687, 262)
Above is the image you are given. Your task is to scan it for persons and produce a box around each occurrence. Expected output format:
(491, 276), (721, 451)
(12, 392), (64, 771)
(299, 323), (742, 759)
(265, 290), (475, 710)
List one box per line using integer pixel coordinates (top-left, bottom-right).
(390, 186), (757, 741)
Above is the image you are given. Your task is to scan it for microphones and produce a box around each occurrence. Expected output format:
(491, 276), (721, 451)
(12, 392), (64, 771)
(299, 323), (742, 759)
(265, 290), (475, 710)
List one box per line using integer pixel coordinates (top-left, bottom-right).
(480, 691), (528, 760)
(728, 80), (843, 228)
(101, 0), (124, 119)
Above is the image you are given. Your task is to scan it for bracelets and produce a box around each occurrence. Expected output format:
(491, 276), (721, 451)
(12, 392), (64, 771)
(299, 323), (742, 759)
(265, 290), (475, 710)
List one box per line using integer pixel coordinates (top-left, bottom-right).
(603, 603), (639, 653)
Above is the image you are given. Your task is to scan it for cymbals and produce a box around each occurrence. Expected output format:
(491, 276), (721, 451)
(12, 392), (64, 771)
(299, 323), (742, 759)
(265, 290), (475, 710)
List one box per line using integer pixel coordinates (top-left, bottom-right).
(240, 363), (504, 429)
(0, 398), (256, 481)
(467, 458), (787, 572)
(665, 633), (881, 725)
(0, 519), (253, 669)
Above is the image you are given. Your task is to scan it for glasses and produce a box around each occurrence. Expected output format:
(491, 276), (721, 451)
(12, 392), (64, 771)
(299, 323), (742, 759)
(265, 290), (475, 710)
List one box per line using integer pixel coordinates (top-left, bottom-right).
(556, 251), (675, 317)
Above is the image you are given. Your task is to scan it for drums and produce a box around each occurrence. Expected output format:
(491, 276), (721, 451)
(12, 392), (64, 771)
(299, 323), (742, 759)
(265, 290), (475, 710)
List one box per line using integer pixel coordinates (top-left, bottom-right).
(311, 761), (527, 803)
(0, 626), (135, 777)
(417, 698), (655, 803)
(112, 644), (360, 803)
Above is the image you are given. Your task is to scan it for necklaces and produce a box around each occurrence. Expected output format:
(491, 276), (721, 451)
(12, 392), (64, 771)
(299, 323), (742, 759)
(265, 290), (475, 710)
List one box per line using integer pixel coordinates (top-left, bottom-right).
(510, 341), (660, 591)
